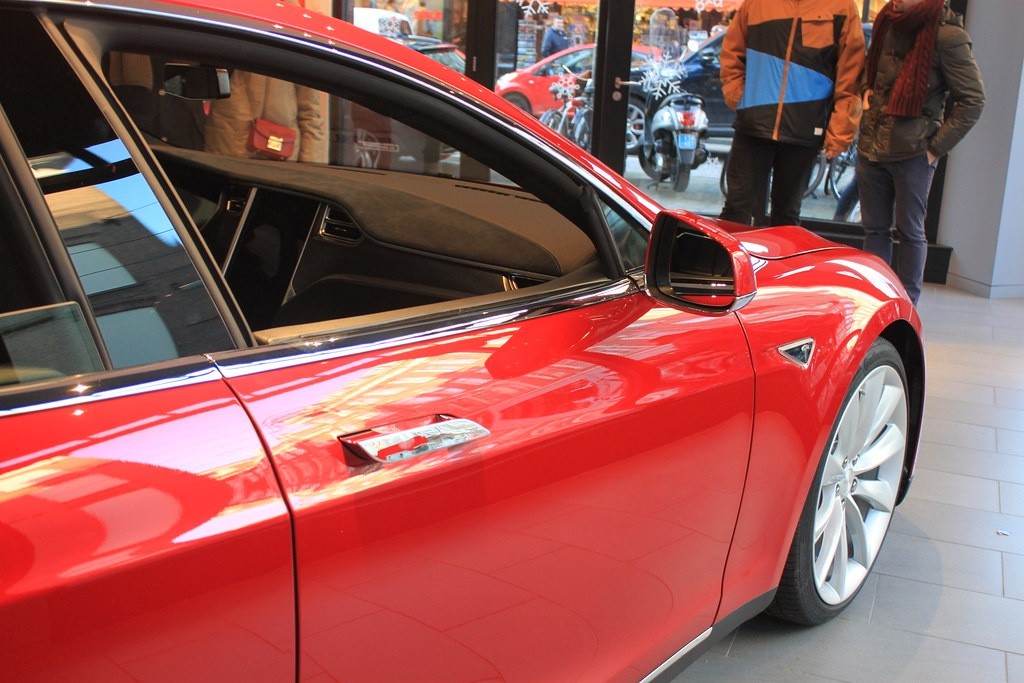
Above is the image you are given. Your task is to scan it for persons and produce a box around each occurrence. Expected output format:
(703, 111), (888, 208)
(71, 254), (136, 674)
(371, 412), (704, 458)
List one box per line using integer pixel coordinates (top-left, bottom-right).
(452, 9), (467, 54)
(856, 0), (986, 309)
(383, 0), (400, 13)
(541, 16), (569, 57)
(720, 0), (866, 227)
(683, 18), (697, 45)
(158, 62), (330, 163)
(418, 2), (427, 11)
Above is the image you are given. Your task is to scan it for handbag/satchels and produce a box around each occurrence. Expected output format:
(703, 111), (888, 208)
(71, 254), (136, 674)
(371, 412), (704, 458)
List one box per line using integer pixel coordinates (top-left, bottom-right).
(246, 118), (298, 161)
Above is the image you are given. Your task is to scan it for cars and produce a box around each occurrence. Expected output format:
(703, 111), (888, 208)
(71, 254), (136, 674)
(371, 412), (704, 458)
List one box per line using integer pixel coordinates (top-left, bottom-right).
(494, 42), (676, 118)
(625, 23), (859, 167)
(1, 0), (929, 683)
(329, 34), (468, 169)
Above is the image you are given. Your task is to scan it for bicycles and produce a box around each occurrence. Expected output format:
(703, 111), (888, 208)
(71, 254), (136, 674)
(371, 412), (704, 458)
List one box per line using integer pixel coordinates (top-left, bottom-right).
(720, 129), (859, 203)
(540, 63), (595, 151)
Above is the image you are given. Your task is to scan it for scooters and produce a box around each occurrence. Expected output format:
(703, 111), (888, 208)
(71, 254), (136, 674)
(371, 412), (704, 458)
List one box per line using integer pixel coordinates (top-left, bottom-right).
(628, 65), (711, 193)
